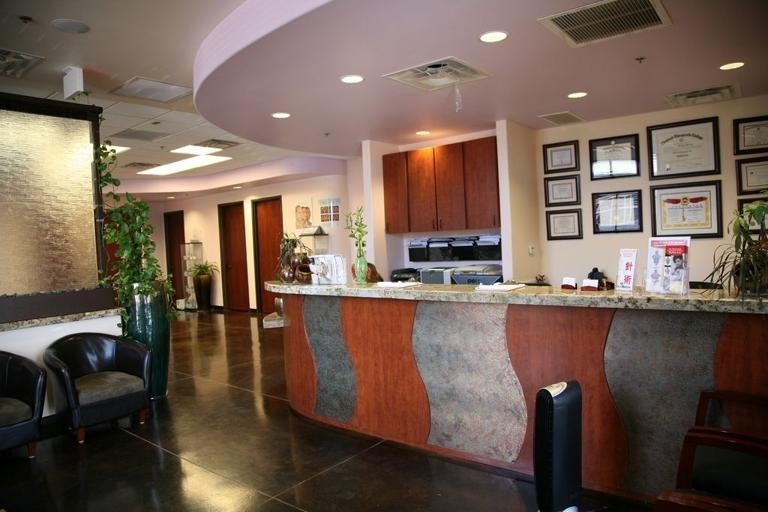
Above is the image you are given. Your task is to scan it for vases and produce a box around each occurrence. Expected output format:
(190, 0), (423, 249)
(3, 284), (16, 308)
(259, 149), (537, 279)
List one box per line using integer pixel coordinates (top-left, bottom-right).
(295, 264), (311, 283)
(356, 247), (367, 283)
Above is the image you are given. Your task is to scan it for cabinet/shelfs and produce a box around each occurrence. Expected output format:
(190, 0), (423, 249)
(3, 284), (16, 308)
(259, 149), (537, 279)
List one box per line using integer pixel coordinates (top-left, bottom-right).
(382, 151), (408, 235)
(463, 136), (500, 228)
(408, 142), (466, 231)
(180, 242), (203, 310)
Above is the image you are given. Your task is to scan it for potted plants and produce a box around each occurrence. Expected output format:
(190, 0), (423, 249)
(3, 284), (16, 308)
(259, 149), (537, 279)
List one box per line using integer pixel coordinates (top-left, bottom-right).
(188, 262), (219, 313)
(98, 115), (170, 400)
(275, 234), (307, 283)
(705, 204), (768, 297)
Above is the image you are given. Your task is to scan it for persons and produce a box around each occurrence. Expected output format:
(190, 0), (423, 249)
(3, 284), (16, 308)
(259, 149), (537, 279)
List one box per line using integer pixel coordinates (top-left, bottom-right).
(671, 255), (684, 275)
(295, 205), (312, 230)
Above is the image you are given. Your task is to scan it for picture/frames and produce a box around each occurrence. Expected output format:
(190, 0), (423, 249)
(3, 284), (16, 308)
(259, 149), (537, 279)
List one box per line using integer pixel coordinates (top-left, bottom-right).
(542, 115), (768, 242)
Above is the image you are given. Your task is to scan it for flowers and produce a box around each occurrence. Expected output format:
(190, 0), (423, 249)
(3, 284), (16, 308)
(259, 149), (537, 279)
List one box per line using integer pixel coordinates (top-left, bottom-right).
(344, 205), (368, 249)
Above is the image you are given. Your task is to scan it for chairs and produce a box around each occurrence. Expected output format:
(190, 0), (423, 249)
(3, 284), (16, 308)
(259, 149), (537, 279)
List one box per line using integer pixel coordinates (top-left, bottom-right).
(0, 351), (47, 459)
(533, 380), (583, 512)
(43, 333), (153, 444)
(657, 390), (768, 511)
